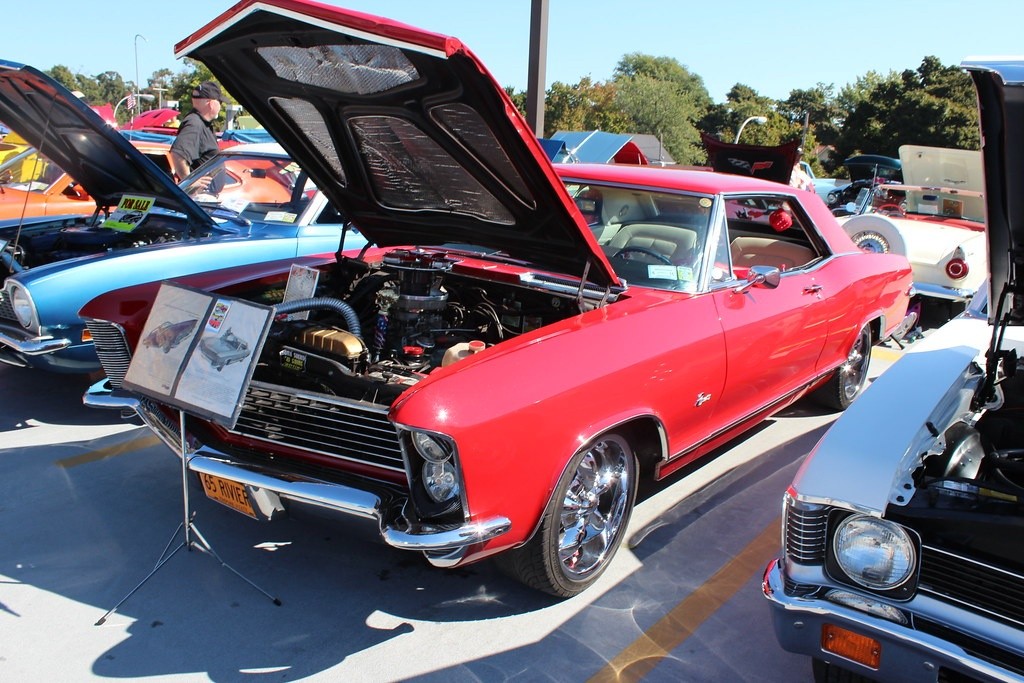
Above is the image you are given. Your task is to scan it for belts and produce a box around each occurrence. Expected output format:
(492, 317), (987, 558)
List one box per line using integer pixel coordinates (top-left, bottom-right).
(185, 188), (218, 197)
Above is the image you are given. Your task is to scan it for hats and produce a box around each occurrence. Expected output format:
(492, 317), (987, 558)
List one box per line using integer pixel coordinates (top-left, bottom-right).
(191, 81), (231, 105)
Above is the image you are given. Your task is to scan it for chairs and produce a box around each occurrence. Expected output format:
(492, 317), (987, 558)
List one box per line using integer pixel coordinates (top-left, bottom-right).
(608, 223), (696, 266)
(727, 237), (811, 270)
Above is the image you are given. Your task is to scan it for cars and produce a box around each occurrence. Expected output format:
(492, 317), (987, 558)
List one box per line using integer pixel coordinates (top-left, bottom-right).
(0, 58), (374, 385)
(871, 145), (986, 309)
(764, 58), (1024, 683)
(800, 161), (851, 207)
(78, 0), (913, 599)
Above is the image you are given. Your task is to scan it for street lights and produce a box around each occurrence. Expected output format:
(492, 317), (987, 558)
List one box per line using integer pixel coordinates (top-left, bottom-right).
(112, 92), (155, 119)
(733, 114), (767, 145)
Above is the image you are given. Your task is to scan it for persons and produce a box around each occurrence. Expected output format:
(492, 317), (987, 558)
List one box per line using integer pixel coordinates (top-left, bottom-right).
(788, 148), (814, 191)
(169, 82), (232, 201)
